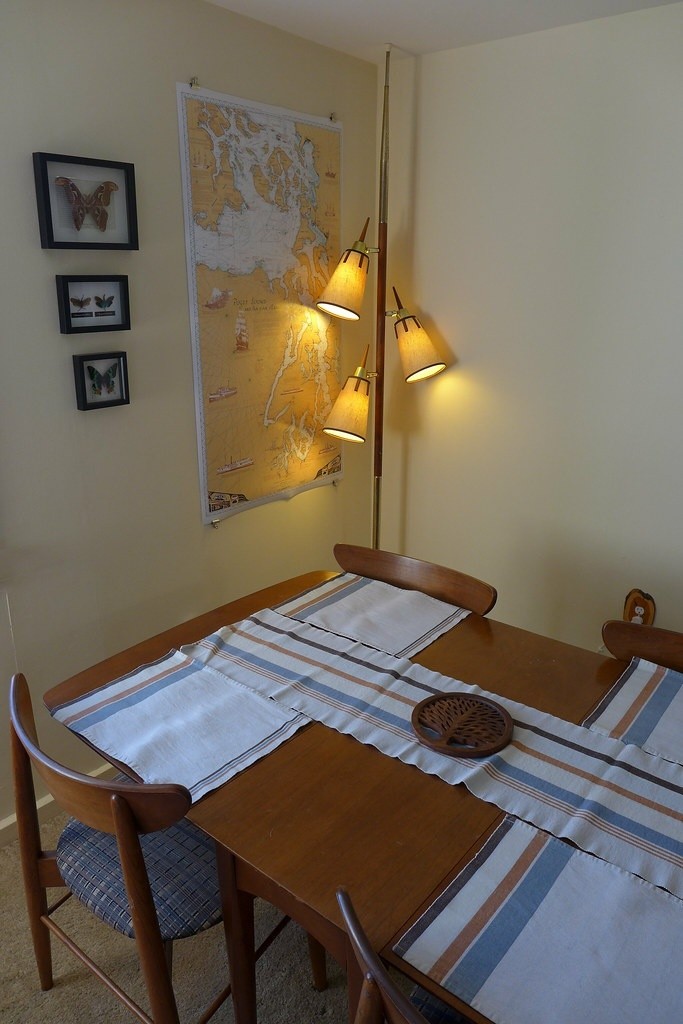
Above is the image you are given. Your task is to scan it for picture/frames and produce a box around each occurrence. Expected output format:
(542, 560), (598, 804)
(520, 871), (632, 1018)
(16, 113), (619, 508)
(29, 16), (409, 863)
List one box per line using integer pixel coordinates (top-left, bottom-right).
(55, 275), (131, 335)
(32, 151), (140, 251)
(73, 350), (131, 411)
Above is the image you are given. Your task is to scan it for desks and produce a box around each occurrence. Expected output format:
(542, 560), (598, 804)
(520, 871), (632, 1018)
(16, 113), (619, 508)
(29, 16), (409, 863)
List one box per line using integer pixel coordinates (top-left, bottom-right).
(44, 571), (683, 1023)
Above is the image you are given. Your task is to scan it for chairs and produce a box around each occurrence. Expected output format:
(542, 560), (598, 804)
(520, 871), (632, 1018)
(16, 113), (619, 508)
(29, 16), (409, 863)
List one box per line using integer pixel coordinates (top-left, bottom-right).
(602, 619), (683, 673)
(333, 542), (497, 620)
(10, 671), (330, 1023)
(333, 886), (431, 1024)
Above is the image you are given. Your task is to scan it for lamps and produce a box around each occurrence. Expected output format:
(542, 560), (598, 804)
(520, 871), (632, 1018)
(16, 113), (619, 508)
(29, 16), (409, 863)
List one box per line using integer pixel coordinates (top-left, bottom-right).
(317, 218), (371, 323)
(385, 286), (446, 385)
(321, 344), (371, 443)
